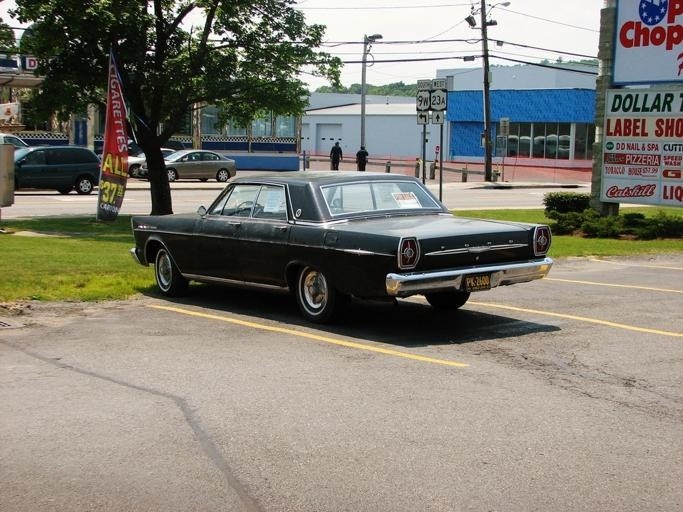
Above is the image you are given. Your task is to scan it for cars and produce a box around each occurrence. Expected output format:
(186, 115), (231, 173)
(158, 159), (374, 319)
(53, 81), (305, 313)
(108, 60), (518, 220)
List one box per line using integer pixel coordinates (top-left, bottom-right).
(0, 133), (236, 194)
(129, 171), (553, 321)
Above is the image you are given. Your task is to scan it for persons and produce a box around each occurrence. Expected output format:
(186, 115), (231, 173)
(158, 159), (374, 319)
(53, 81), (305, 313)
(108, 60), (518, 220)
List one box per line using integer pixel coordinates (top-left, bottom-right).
(46, 110), (59, 132)
(58, 114), (70, 132)
(354, 145), (369, 172)
(329, 142), (343, 171)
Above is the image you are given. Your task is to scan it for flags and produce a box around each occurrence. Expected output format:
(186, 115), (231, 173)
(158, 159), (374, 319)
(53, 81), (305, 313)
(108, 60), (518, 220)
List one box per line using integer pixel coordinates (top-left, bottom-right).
(94, 44), (130, 222)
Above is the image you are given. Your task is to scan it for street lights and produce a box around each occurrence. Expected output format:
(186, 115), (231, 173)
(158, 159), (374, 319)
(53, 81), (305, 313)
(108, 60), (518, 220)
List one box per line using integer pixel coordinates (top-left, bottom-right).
(361, 34), (383, 149)
(465, 1), (511, 181)
